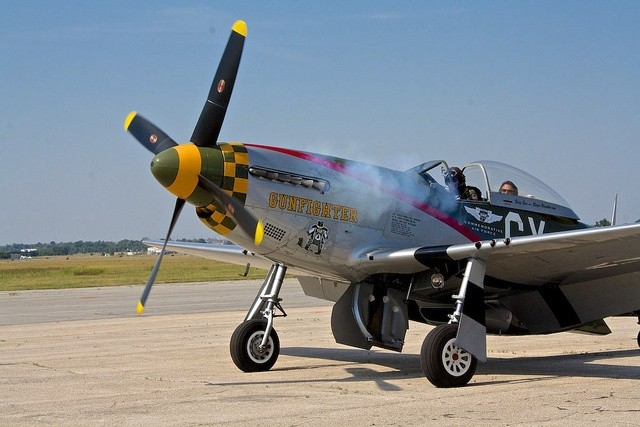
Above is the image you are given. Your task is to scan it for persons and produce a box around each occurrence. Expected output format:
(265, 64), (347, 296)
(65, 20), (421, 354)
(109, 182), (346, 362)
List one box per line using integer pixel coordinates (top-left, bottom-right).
(467, 186), (482, 199)
(500, 180), (518, 195)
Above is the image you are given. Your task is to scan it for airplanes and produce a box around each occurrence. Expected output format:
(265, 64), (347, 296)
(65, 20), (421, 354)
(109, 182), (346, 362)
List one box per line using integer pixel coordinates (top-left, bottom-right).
(123, 20), (640, 388)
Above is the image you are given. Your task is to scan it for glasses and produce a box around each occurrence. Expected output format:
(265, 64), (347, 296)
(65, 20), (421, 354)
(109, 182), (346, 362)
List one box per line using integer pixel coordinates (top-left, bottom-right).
(500, 189), (515, 193)
